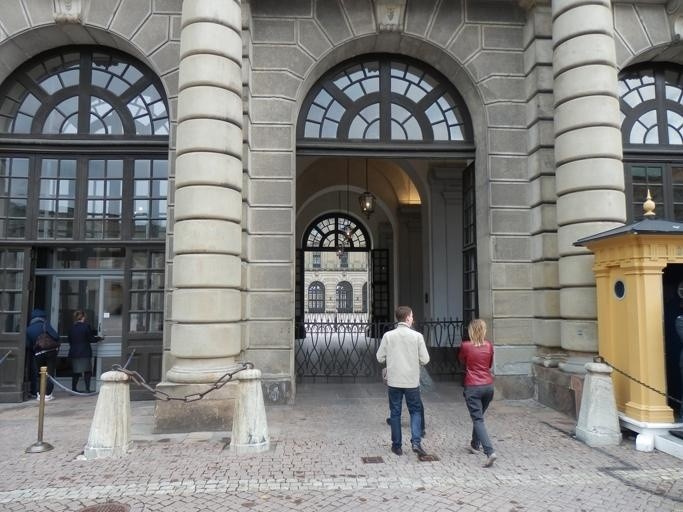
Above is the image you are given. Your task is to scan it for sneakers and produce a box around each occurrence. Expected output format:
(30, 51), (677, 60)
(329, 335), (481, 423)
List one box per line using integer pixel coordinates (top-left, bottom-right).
(486, 455), (496, 466)
(36, 394), (54, 402)
(391, 446), (402, 454)
(413, 445), (426, 455)
(469, 447), (479, 454)
(386, 417), (390, 425)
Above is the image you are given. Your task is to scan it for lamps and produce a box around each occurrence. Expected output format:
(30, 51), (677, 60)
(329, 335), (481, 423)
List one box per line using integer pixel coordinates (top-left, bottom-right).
(359, 158), (377, 220)
(336, 175), (344, 259)
(344, 158), (353, 241)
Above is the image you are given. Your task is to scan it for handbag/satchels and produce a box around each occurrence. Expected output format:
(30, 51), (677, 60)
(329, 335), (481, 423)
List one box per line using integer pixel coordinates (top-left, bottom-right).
(36, 335), (58, 349)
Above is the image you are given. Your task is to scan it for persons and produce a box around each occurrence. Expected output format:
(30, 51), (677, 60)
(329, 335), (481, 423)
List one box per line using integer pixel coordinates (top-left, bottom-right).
(457, 319), (498, 469)
(376, 305), (431, 457)
(381, 322), (433, 438)
(27, 308), (61, 401)
(66, 310), (105, 393)
